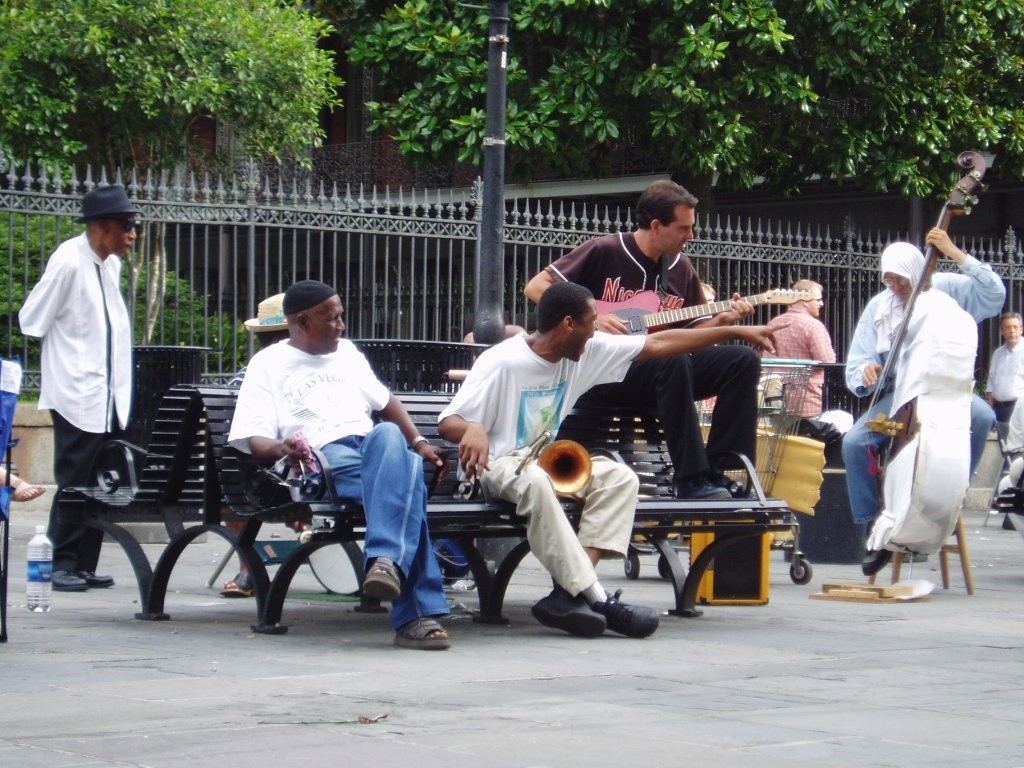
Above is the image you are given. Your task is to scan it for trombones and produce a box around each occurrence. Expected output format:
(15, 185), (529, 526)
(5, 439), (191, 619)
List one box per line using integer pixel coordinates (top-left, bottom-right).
(516, 431), (591, 494)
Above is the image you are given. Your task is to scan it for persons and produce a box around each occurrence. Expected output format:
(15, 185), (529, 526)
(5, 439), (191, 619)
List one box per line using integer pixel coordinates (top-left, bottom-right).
(986, 312), (1024, 458)
(220, 292), (289, 596)
(437, 280), (791, 637)
(0, 466), (47, 504)
(840, 227), (1006, 575)
(525, 178), (762, 498)
(227, 280), (451, 650)
(16, 186), (144, 595)
(761, 280), (836, 429)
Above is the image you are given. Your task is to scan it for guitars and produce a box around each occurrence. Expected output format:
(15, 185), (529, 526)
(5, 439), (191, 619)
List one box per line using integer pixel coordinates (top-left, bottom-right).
(592, 290), (823, 337)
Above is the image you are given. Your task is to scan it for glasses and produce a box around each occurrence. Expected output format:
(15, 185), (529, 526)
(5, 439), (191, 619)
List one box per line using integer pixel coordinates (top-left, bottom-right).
(107, 217), (135, 232)
(881, 276), (910, 287)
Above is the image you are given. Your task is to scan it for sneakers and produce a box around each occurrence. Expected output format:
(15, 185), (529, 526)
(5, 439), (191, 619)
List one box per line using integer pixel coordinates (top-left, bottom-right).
(588, 589), (660, 639)
(532, 584), (607, 637)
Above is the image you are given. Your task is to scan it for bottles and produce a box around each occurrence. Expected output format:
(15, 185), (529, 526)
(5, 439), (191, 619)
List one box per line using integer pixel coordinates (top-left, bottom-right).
(26, 526), (53, 612)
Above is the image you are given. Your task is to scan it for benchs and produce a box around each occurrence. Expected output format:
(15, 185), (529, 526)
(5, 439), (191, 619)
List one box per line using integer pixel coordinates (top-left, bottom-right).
(46, 382), (798, 633)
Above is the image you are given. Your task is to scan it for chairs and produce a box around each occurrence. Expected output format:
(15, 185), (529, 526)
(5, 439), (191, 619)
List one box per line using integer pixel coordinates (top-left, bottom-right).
(0, 357), (25, 644)
(981, 419), (1024, 536)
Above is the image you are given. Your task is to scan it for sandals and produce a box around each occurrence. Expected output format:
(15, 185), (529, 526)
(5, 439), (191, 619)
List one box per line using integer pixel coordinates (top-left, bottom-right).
(395, 617), (450, 650)
(363, 557), (401, 601)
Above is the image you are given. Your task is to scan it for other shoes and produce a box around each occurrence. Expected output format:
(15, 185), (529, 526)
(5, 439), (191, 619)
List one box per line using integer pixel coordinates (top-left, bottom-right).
(219, 571), (255, 597)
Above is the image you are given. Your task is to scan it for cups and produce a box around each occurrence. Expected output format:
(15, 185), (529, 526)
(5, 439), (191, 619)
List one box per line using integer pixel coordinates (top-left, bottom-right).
(757, 373), (786, 413)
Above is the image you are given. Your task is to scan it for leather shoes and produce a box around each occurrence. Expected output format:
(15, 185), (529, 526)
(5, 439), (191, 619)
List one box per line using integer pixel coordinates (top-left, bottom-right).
(676, 475), (732, 499)
(713, 473), (744, 499)
(861, 521), (893, 576)
(52, 570), (88, 591)
(73, 569), (115, 589)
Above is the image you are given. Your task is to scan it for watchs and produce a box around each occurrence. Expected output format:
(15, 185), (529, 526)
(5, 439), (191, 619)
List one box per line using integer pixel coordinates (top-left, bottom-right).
(410, 435), (429, 449)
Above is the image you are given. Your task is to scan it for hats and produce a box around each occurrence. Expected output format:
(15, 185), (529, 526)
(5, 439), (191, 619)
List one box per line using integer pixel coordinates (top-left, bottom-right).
(72, 185), (143, 224)
(244, 292), (288, 333)
(282, 279), (336, 314)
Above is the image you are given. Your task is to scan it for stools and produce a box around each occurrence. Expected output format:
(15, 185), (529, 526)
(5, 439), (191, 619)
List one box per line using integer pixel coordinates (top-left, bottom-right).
(864, 510), (974, 595)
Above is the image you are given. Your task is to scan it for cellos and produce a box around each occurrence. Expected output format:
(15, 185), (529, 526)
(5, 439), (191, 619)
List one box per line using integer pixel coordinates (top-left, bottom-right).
(867, 150), (989, 582)
